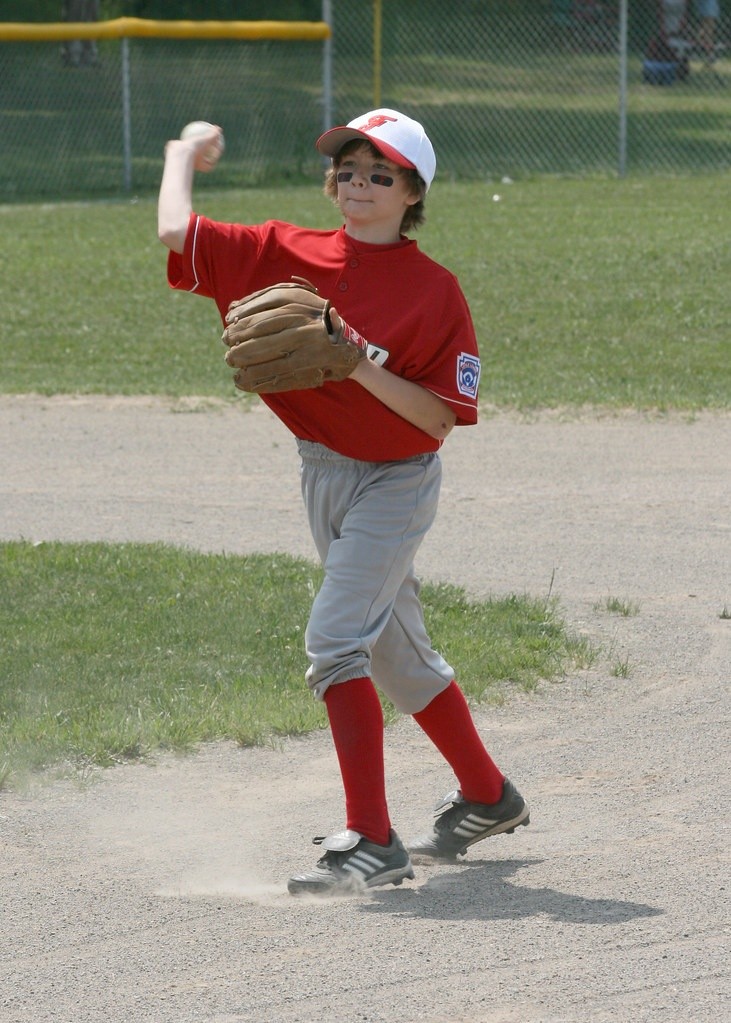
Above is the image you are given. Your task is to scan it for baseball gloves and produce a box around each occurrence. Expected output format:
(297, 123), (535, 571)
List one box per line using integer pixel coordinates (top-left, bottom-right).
(219, 281), (373, 396)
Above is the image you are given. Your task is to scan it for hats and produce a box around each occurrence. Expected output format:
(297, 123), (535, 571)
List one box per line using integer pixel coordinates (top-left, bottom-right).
(316, 109), (436, 194)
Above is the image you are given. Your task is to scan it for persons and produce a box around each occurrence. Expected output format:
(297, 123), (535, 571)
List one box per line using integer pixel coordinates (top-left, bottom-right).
(657, 0), (691, 80)
(157, 108), (532, 897)
(60, 0), (99, 68)
(698, 0), (719, 41)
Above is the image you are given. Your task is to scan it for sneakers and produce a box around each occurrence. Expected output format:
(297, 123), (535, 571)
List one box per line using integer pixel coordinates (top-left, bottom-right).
(287, 828), (415, 897)
(405, 776), (531, 864)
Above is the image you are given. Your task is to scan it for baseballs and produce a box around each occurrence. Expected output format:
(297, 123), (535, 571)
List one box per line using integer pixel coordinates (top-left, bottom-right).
(179, 120), (227, 165)
